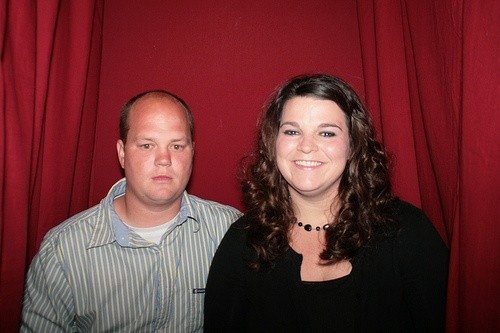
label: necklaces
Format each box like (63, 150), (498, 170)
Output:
(290, 217), (337, 232)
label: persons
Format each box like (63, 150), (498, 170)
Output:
(202, 74), (449, 333)
(19, 90), (245, 333)
